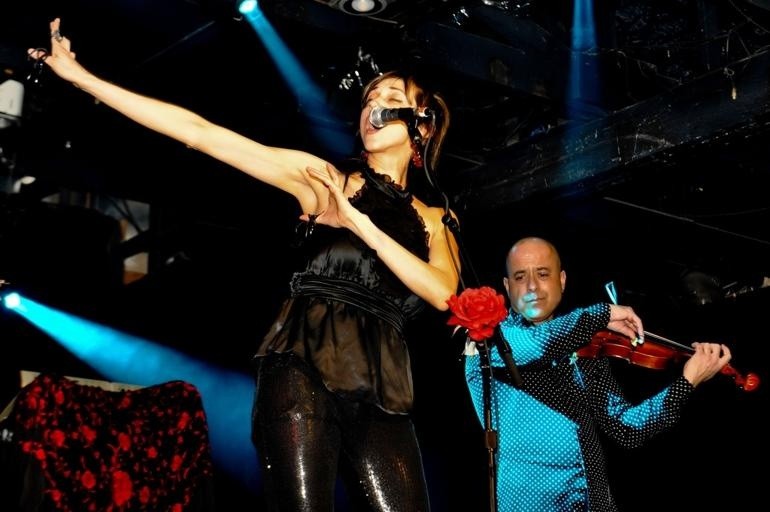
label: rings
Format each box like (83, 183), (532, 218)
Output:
(50, 29), (62, 43)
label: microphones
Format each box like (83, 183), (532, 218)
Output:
(369, 106), (432, 128)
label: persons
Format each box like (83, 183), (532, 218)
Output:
(462, 235), (734, 511)
(24, 16), (462, 509)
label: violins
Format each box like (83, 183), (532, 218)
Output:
(577, 329), (761, 391)
